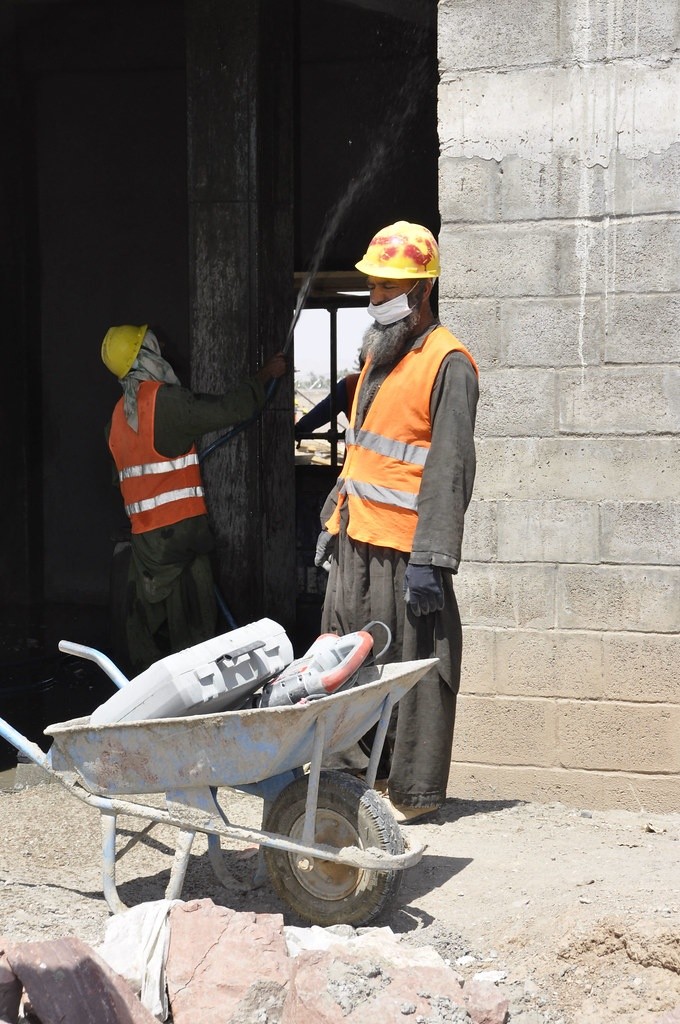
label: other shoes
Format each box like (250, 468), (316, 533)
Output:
(386, 801), (442, 822)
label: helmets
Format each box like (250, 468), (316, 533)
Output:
(355, 221), (441, 278)
(100, 325), (150, 380)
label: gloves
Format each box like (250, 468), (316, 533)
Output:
(404, 562), (444, 615)
(313, 529), (336, 572)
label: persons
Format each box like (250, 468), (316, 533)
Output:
(313, 218), (481, 827)
(99, 322), (293, 682)
(295, 348), (367, 461)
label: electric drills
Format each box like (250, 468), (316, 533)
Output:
(232, 632), (374, 708)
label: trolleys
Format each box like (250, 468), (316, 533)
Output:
(0, 639), (443, 928)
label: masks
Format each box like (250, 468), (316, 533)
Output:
(366, 282), (422, 325)
